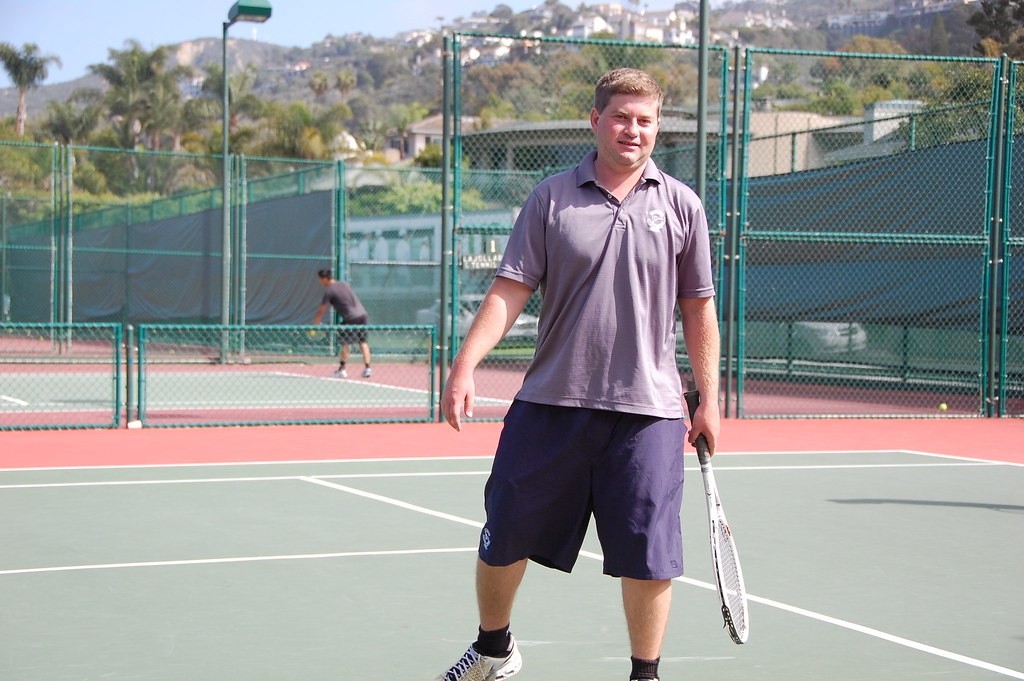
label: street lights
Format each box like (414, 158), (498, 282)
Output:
(217, 0), (274, 364)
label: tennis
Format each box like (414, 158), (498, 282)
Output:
(939, 402), (948, 412)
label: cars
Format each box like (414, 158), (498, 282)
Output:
(674, 319), (688, 353)
(780, 321), (869, 358)
(415, 293), (538, 341)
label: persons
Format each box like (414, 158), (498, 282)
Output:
(310, 268), (372, 378)
(437, 68), (721, 681)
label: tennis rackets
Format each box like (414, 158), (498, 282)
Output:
(683, 389), (750, 645)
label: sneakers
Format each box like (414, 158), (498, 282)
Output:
(433, 631), (522, 681)
(361, 368), (371, 377)
(334, 369), (347, 378)
